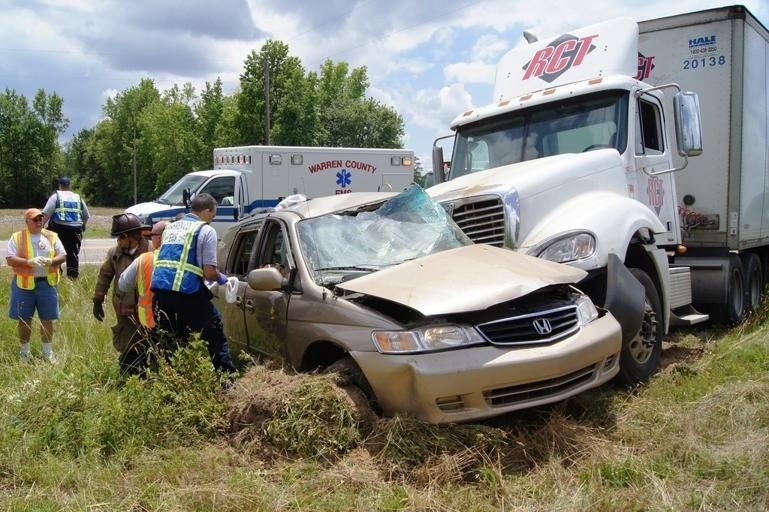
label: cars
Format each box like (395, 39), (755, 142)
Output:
(209, 180), (634, 438)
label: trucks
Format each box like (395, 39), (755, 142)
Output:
(422, 160), (489, 194)
(415, 1), (769, 384)
(117, 143), (416, 244)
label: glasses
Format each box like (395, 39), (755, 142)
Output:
(120, 233), (128, 239)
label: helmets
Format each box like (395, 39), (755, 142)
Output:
(111, 214), (152, 235)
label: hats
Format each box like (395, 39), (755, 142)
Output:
(58, 177), (70, 184)
(24, 207), (44, 219)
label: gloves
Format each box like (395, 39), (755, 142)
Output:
(28, 256), (52, 268)
(218, 272), (227, 284)
(93, 297), (104, 321)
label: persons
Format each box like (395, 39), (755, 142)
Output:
(118, 219), (192, 374)
(4, 206), (68, 363)
(92, 213), (156, 383)
(148, 192), (241, 391)
(41, 175), (91, 281)
